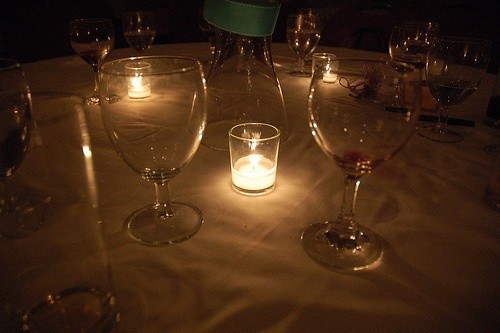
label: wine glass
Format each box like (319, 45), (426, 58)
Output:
(70, 17), (122, 107)
(380, 21), (487, 142)
(99, 55), (207, 247)
(285, 7), (323, 76)
(1, 58), (52, 216)
(301, 58), (421, 273)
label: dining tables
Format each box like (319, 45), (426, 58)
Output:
(0, 41), (500, 333)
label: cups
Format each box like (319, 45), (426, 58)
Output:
(189, 0), (290, 151)
(23, 284), (121, 333)
(124, 62), (152, 100)
(229, 122), (281, 196)
(123, 12), (155, 53)
(312, 53), (338, 83)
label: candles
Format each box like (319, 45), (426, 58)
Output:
(128, 76), (151, 98)
(233, 153), (277, 190)
(323, 64), (337, 82)
